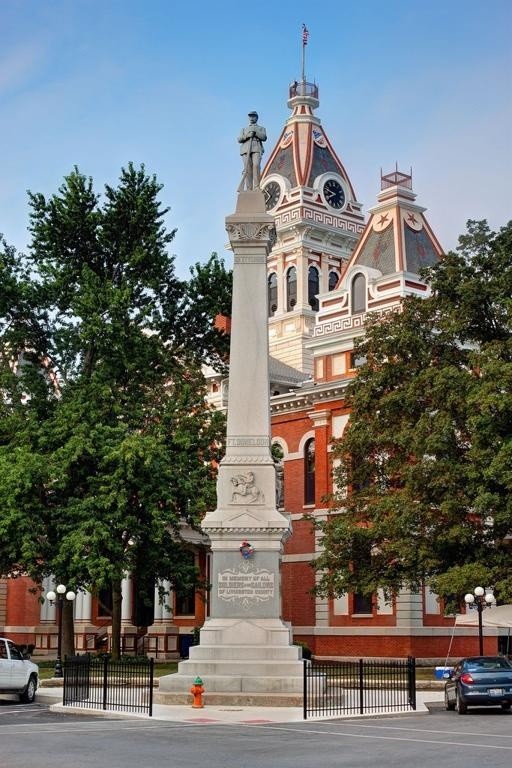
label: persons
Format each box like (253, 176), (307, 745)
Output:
(240, 470), (254, 496)
(238, 110), (267, 191)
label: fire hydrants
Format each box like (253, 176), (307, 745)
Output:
(190, 676), (205, 709)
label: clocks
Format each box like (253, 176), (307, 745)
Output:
(323, 178), (346, 209)
(260, 179), (281, 211)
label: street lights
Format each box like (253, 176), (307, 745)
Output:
(45, 585), (75, 678)
(465, 586), (494, 656)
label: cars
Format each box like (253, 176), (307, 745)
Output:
(444, 655), (512, 714)
(0, 636), (40, 702)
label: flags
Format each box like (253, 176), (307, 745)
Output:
(313, 131), (324, 144)
(302, 26), (309, 45)
